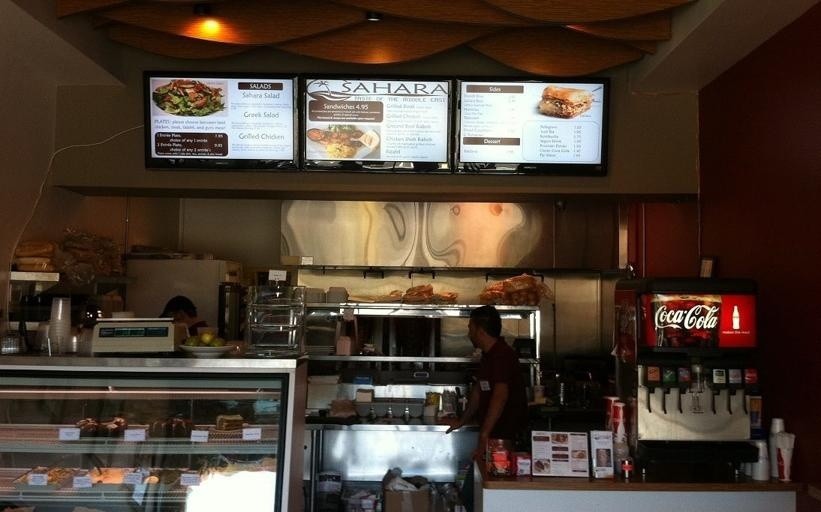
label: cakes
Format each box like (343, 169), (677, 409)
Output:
(75, 417), (128, 438)
(216, 414), (244, 430)
(144, 412), (193, 438)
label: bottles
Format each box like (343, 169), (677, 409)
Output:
(490, 439), (511, 477)
(361, 343), (375, 356)
(440, 483), (471, 512)
(731, 305), (740, 330)
(317, 470), (382, 512)
(429, 482), (437, 511)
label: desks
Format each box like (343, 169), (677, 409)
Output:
(482, 472), (801, 512)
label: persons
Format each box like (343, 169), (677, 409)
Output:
(159, 294), (226, 471)
(445, 305), (528, 511)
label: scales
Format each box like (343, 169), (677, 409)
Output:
(91, 317), (191, 357)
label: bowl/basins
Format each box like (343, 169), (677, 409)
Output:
(303, 287), (348, 304)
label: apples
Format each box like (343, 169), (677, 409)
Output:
(185, 331), (226, 347)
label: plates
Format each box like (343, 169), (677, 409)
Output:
(304, 345), (334, 355)
(309, 125), (379, 160)
(178, 345), (237, 357)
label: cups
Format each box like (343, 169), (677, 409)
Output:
(48, 296), (91, 355)
(741, 418), (796, 483)
(605, 396), (632, 480)
(650, 293), (722, 347)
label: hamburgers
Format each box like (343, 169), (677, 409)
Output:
(538, 88), (590, 118)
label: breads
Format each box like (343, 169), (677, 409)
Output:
(482, 276), (535, 305)
(392, 284), (459, 304)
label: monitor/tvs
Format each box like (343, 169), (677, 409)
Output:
(453, 75), (611, 176)
(142, 69), (300, 172)
(298, 71), (455, 175)
